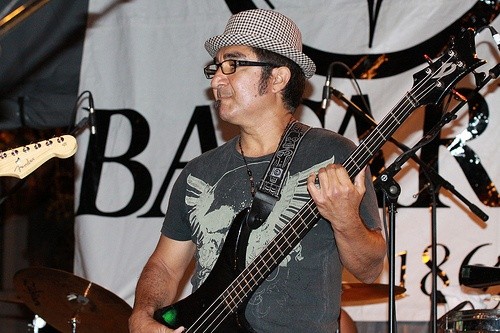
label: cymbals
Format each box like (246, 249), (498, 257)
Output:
(12, 267), (134, 333)
(336, 282), (406, 306)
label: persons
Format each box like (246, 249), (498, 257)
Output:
(129, 9), (386, 333)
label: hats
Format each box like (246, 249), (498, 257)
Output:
(204, 8), (317, 79)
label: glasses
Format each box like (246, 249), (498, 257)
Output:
(204, 60), (284, 79)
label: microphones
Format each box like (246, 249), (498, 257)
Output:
(89, 93), (97, 134)
(321, 66), (332, 109)
(437, 302), (468, 329)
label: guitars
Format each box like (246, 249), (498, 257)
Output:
(1, 132), (77, 181)
(152, 28), (487, 332)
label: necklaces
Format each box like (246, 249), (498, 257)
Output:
(238, 134), (254, 197)
